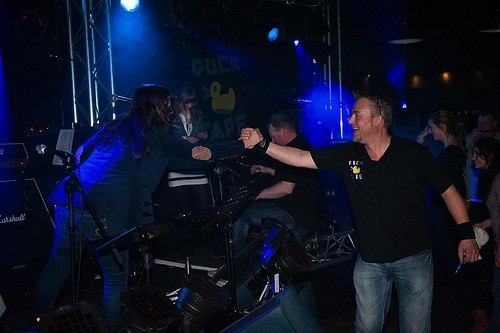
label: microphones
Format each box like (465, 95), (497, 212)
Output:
(110, 94), (133, 104)
(36, 144), (73, 157)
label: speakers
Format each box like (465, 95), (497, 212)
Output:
(220, 285), (324, 333)
(0, 177), (56, 272)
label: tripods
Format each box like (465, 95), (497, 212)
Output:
(304, 192), (357, 264)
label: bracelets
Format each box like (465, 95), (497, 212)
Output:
(253, 138), (269, 154)
(455, 222), (476, 241)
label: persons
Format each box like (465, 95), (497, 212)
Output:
(417, 108), (500, 333)
(163, 100), (214, 221)
(19, 83), (264, 333)
(214, 111), (324, 270)
(240, 91), (481, 333)
